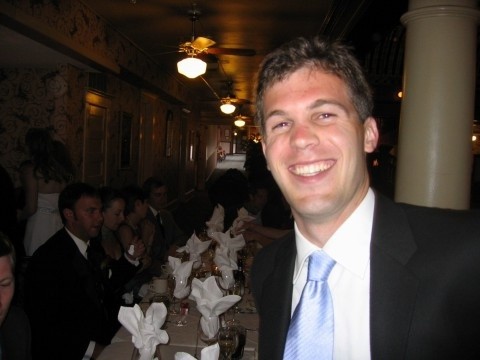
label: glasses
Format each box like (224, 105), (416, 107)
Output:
(152, 192), (169, 197)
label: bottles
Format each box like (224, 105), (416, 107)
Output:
(235, 258), (245, 285)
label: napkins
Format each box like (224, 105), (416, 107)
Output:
(117, 301), (170, 360)
(167, 202), (256, 337)
(173, 342), (221, 360)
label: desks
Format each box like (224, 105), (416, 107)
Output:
(109, 240), (258, 350)
(98, 343), (260, 360)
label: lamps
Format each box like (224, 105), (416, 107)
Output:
(177, 57), (207, 78)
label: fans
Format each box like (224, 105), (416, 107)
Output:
(207, 79), (255, 121)
(150, 7), (257, 57)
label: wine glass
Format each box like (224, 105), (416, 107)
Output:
(218, 327), (239, 360)
(225, 283), (241, 322)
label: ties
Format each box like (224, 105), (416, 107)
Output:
(156, 213), (166, 239)
(282, 251), (337, 360)
(87, 245), (96, 264)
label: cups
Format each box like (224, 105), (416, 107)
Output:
(230, 325), (246, 360)
(148, 293), (172, 310)
(149, 279), (168, 293)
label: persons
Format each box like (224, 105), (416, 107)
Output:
(0, 231), (33, 360)
(249, 35), (480, 360)
(222, 168), (295, 239)
(19, 127), (72, 258)
(26, 180), (139, 360)
(95, 176), (186, 300)
